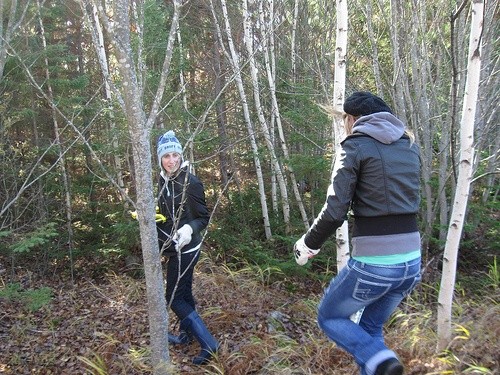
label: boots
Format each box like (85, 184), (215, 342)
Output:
(168, 301), (198, 345)
(182, 311), (220, 365)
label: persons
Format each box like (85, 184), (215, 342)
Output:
(294, 91), (422, 375)
(131, 130), (221, 366)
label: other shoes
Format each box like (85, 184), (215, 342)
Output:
(376, 358), (403, 375)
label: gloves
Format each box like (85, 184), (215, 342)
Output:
(292, 234), (321, 266)
(172, 225), (192, 252)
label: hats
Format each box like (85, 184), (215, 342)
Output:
(344, 91), (393, 116)
(157, 130), (184, 198)
